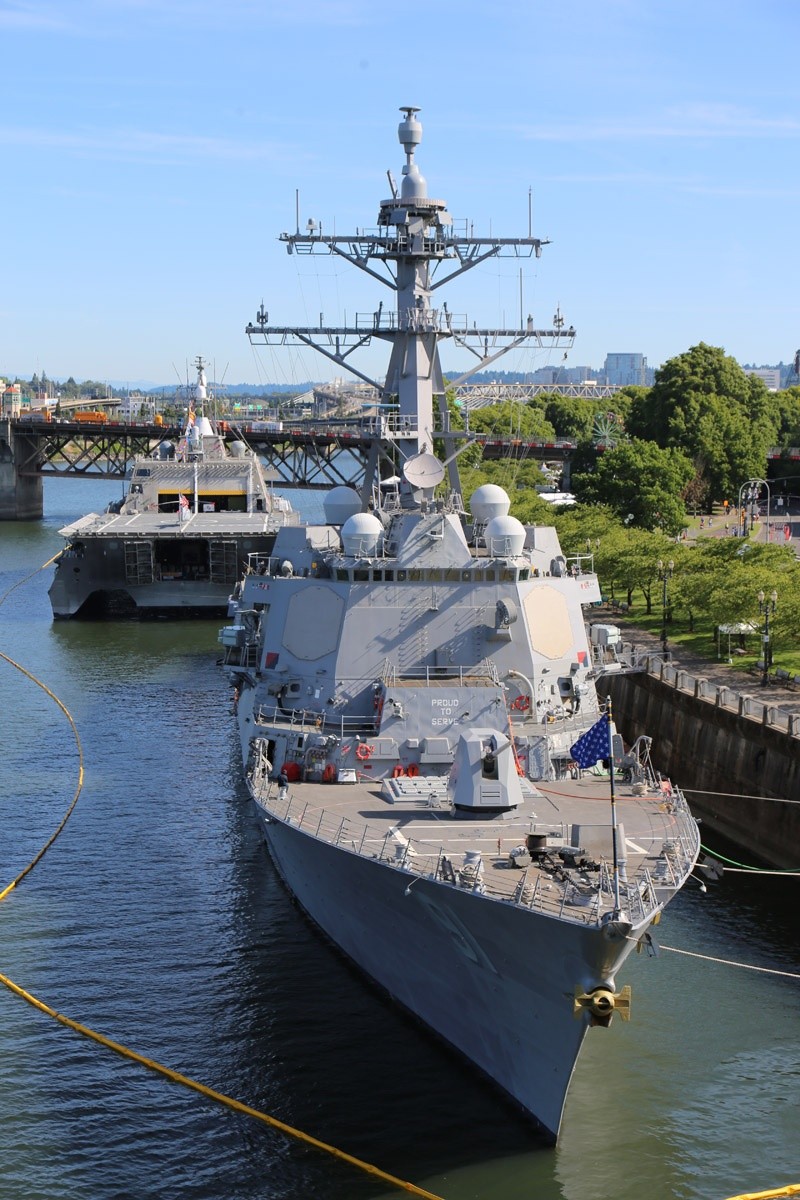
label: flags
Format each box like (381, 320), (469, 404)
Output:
(570, 713), (611, 769)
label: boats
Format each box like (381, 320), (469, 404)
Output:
(42, 354), (301, 620)
(221, 101), (707, 1138)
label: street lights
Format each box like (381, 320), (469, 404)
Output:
(657, 558), (676, 661)
(757, 590), (782, 688)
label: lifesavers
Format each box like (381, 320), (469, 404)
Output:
(356, 745), (371, 760)
(516, 695), (529, 710)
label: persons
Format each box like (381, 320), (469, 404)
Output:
(277, 769), (289, 793)
